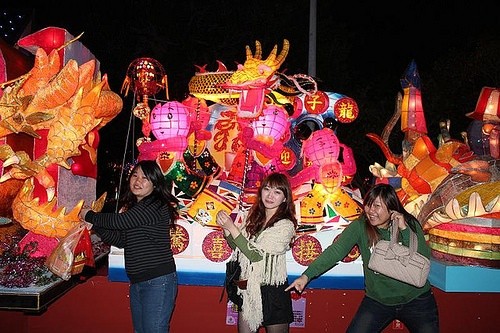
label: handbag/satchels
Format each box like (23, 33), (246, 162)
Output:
(226, 258), (245, 307)
(367, 213), (430, 289)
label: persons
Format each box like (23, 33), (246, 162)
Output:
(216, 172), (297, 333)
(79, 159), (180, 333)
(286, 184), (440, 333)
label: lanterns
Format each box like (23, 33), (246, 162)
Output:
(0, 26), (500, 271)
